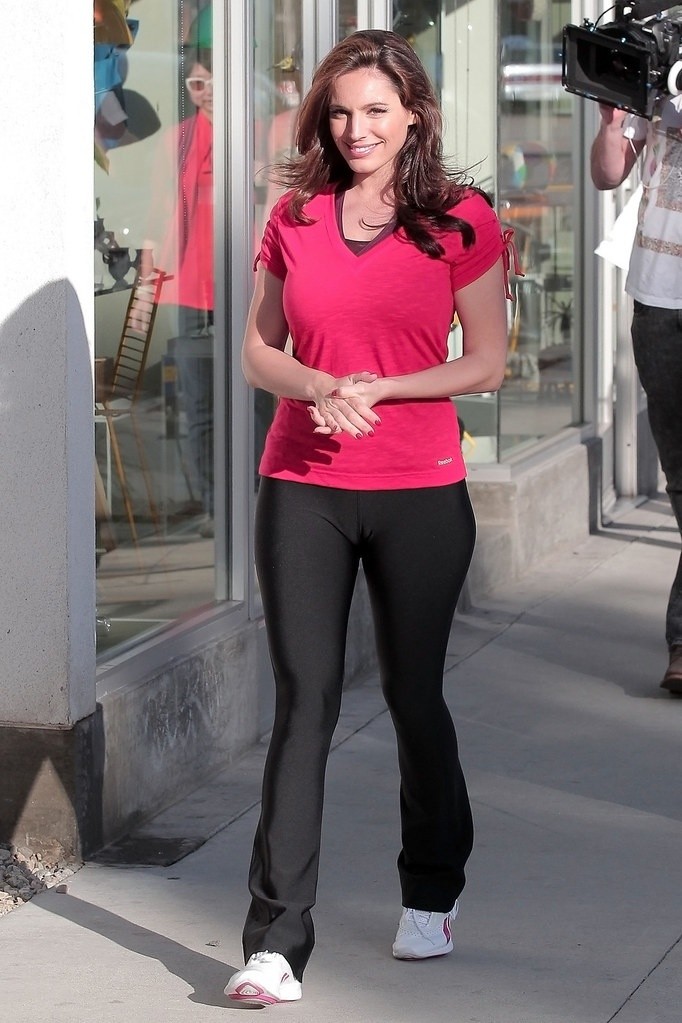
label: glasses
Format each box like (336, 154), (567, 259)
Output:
(185, 78), (214, 93)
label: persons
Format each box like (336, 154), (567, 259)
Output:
(223, 29), (508, 1007)
(128, 44), (265, 541)
(590, 0), (682, 696)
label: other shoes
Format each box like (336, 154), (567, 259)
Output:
(660, 644), (682, 691)
(199, 516), (214, 537)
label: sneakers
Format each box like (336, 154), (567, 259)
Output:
(392, 898), (459, 959)
(224, 951), (302, 1004)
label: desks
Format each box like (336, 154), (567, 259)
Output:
(500, 185), (578, 362)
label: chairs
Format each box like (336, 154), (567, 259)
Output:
(96, 266), (169, 556)
